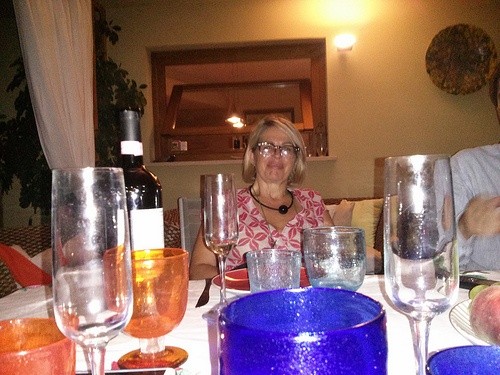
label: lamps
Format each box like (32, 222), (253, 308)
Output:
(224, 63), (246, 128)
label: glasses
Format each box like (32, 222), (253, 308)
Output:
(257, 141), (300, 158)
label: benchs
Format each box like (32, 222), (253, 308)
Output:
(0, 196), (386, 299)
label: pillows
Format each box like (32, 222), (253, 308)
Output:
(352, 198), (384, 248)
(332, 200), (355, 226)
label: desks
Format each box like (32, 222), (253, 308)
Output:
(75, 275), (474, 375)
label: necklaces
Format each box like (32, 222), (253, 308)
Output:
(249, 186), (293, 214)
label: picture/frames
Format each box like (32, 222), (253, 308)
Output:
(244, 107), (295, 125)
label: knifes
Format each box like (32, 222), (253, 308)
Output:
(195, 275), (212, 309)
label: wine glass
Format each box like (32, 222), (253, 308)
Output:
(50, 163), (135, 375)
(114, 244), (192, 369)
(202, 172), (236, 323)
(382, 150), (462, 375)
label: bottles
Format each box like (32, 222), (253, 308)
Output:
(112, 100), (165, 321)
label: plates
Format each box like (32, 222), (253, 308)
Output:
(425, 344), (500, 374)
(457, 268), (500, 291)
(211, 266), (328, 293)
(449, 294), (493, 348)
(423, 20), (495, 95)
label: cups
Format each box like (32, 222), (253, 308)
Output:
(307, 132), (328, 158)
(217, 286), (390, 375)
(246, 249), (302, 295)
(0, 315), (78, 375)
(302, 225), (365, 291)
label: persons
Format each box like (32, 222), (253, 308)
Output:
(435, 72), (499, 272)
(189, 118), (341, 281)
